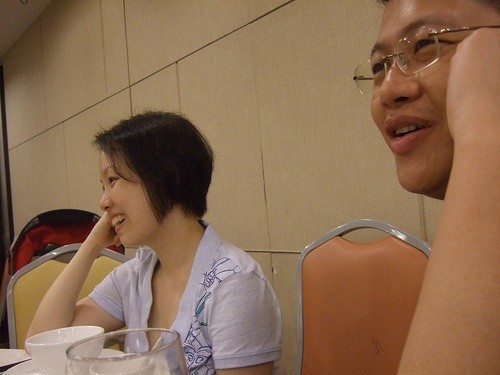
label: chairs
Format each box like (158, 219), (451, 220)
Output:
(295, 219), (431, 375)
(7, 242), (130, 349)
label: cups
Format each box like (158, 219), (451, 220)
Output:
(65, 328), (188, 375)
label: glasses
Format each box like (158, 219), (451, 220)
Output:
(353, 25), (500, 98)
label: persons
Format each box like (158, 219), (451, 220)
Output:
(25, 111), (283, 375)
(352, 0), (500, 375)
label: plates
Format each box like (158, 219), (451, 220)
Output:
(0, 348), (125, 375)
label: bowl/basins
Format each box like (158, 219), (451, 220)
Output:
(25, 326), (104, 375)
